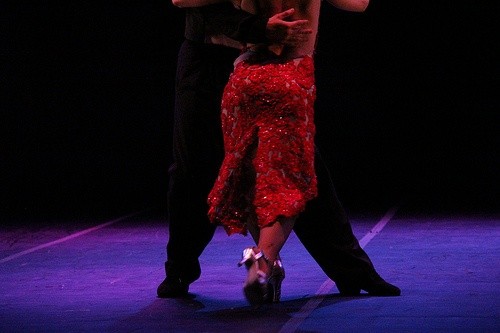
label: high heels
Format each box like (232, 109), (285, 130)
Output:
(237, 248), (267, 312)
(269, 259), (285, 307)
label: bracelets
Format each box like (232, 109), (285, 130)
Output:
(261, 15), (268, 31)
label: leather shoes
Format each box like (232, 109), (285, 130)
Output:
(157, 275), (188, 300)
(336, 280), (402, 297)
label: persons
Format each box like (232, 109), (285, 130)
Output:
(170, 0), (369, 307)
(157, 0), (401, 298)
